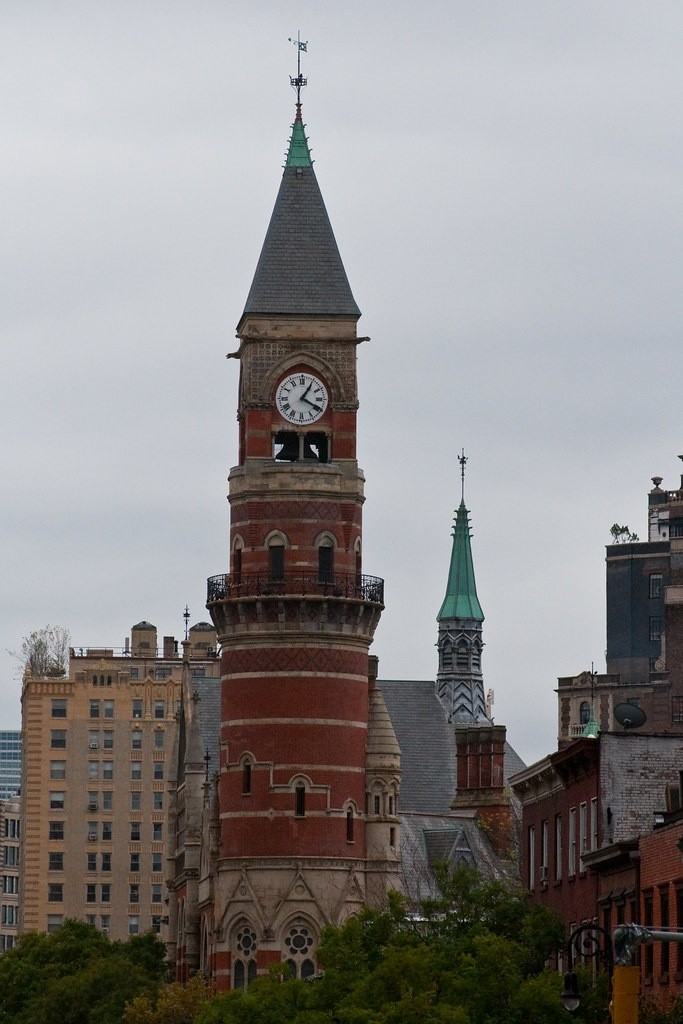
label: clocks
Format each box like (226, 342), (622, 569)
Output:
(275, 373), (328, 425)
